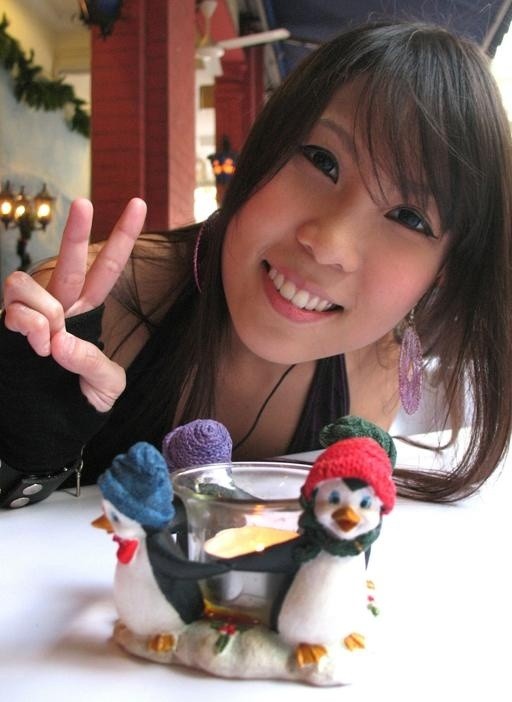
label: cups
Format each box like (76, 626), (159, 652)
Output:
(170, 462), (314, 629)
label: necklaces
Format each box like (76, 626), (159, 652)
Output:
(203, 362), (300, 469)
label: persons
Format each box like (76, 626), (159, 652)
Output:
(0, 18), (509, 515)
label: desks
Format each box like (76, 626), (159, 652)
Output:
(0, 424), (512, 702)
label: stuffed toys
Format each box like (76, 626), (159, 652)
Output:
(319, 416), (397, 618)
(91, 442), (230, 654)
(217, 437), (397, 666)
(161, 419), (246, 561)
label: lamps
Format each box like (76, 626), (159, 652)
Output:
(206, 129), (237, 182)
(0, 178), (64, 274)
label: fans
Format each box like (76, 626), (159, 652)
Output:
(195, 0), (291, 77)
